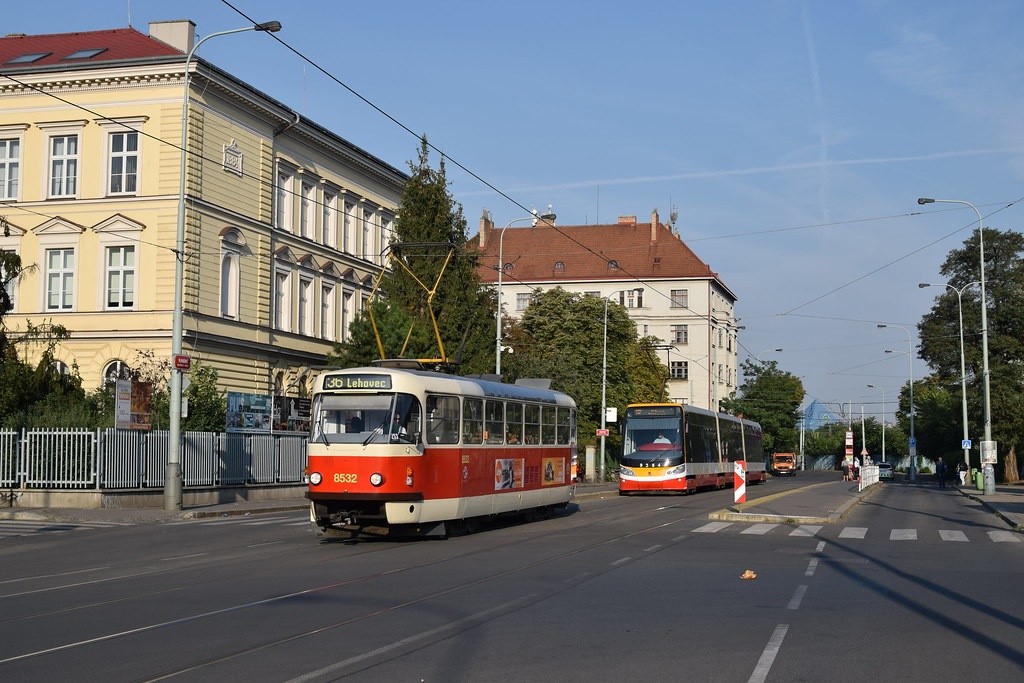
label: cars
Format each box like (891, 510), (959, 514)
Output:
(875, 462), (895, 481)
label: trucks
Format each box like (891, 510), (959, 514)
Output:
(770, 452), (797, 477)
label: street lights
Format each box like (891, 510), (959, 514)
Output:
(599, 288), (645, 483)
(866, 384), (886, 463)
(878, 324), (916, 482)
(756, 349), (782, 384)
(165, 21), (282, 514)
(919, 278), (1000, 486)
(495, 212), (557, 376)
(919, 197), (996, 497)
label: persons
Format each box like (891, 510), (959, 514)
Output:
(383, 413), (406, 434)
(653, 431), (671, 443)
(350, 416), (364, 432)
(936, 457), (948, 487)
(957, 459), (968, 486)
(841, 456), (874, 481)
(506, 425), (521, 444)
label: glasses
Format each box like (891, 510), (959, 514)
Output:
(394, 418), (399, 420)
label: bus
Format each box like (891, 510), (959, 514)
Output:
(303, 358), (578, 542)
(616, 402), (767, 496)
